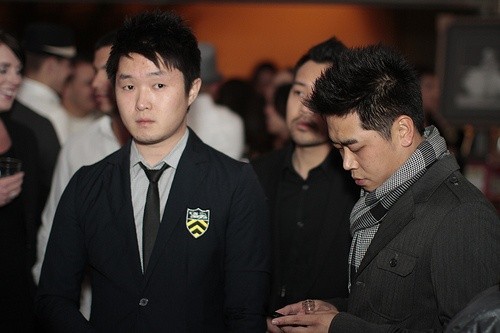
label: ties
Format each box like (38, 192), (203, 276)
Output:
(138, 161), (171, 273)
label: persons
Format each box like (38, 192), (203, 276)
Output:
(268, 43), (500, 333)
(0, 26), (500, 333)
(34, 13), (267, 333)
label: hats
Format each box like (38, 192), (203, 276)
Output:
(33, 24), (76, 58)
